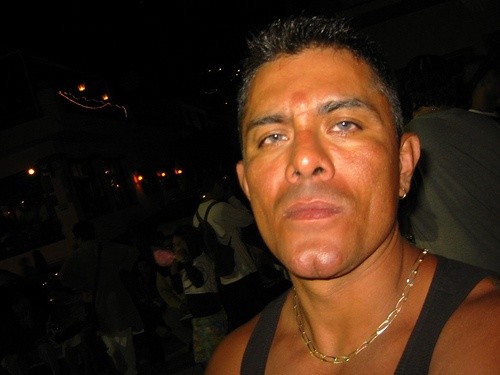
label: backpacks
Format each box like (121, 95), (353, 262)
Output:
(196, 199), (235, 271)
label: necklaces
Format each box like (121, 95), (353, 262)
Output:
(275, 244), (430, 368)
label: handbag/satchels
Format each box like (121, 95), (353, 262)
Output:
(74, 301), (101, 332)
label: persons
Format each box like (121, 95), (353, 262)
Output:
(0, 160), (274, 375)
(197, 17), (500, 375)
